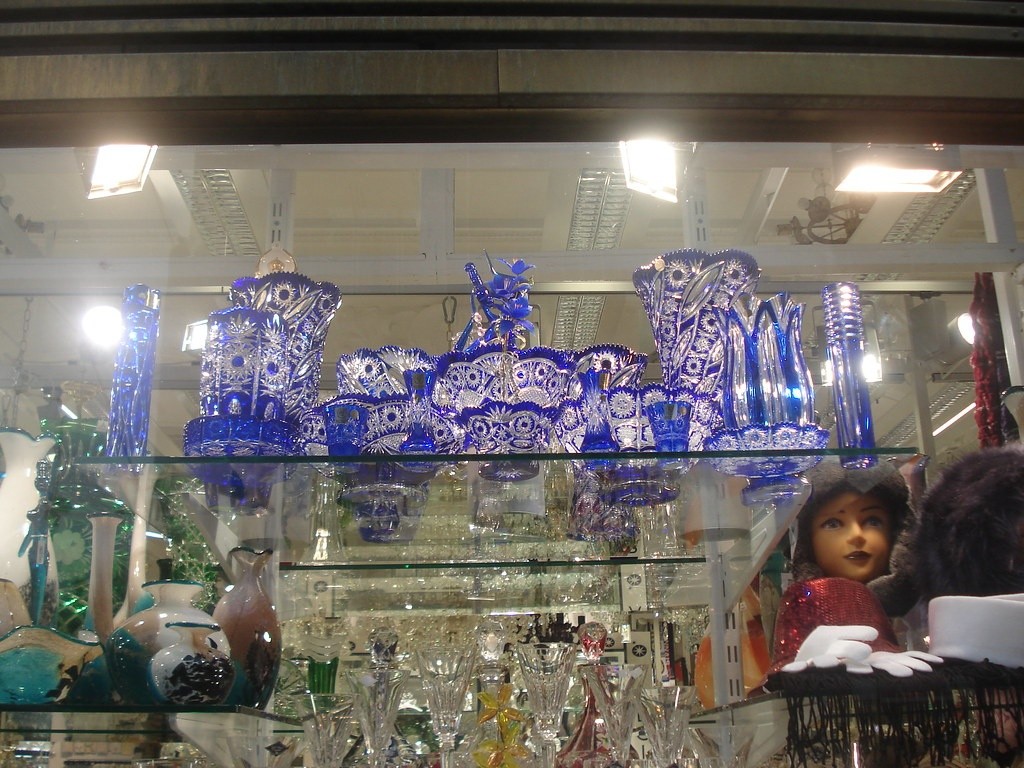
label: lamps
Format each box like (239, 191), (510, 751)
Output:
(71, 144), (158, 201)
(181, 316), (207, 354)
(621, 137), (699, 204)
(81, 294), (129, 350)
(831, 142), (962, 195)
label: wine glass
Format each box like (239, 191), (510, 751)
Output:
(226, 640), (696, 768)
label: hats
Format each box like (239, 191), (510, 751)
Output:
(767, 577), (904, 674)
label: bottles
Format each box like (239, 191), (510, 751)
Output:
(66, 462), (282, 713)
(0, 427), (63, 633)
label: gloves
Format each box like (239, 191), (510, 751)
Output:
(781, 625), (878, 674)
(870, 651), (945, 678)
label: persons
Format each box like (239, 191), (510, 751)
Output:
(805, 477), (902, 580)
